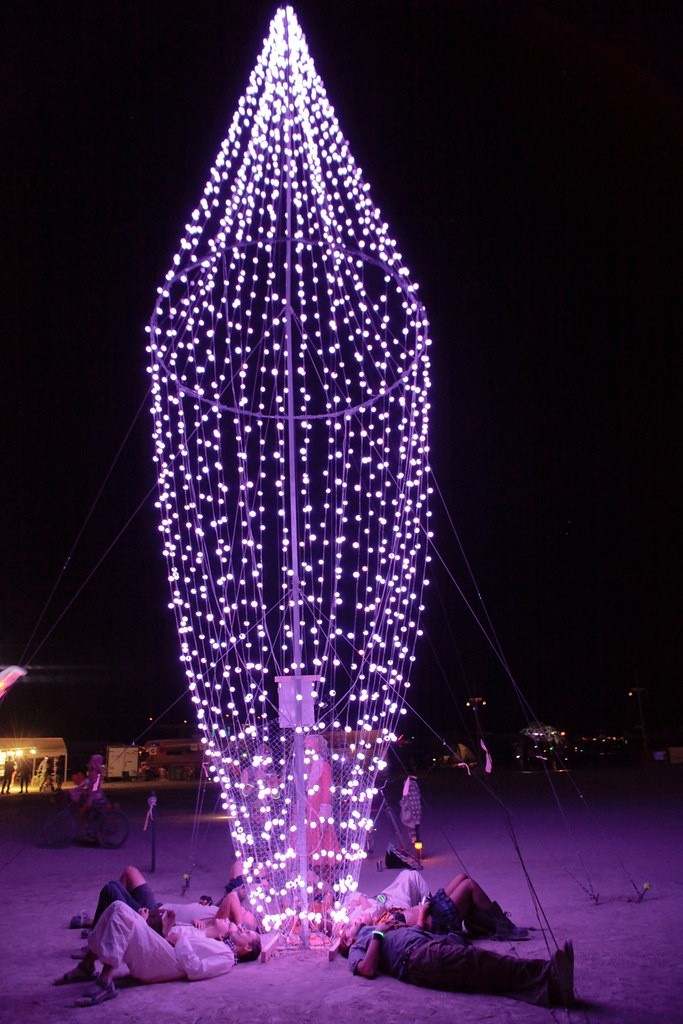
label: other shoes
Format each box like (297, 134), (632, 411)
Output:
(69, 908), (89, 929)
(80, 928), (92, 938)
(549, 937), (575, 1007)
(70, 944), (87, 958)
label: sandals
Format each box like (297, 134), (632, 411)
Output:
(74, 978), (119, 1007)
(63, 962), (99, 982)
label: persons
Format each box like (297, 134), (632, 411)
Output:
(54, 733), (574, 1012)
(2, 756), (105, 842)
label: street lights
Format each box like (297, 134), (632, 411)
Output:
(627, 686), (650, 757)
(465, 696), (488, 757)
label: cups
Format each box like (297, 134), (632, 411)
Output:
(377, 860), (382, 872)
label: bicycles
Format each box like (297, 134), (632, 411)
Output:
(43, 786), (131, 849)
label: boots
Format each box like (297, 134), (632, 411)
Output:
(482, 901), (532, 940)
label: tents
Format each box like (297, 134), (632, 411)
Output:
(0, 737), (67, 785)
